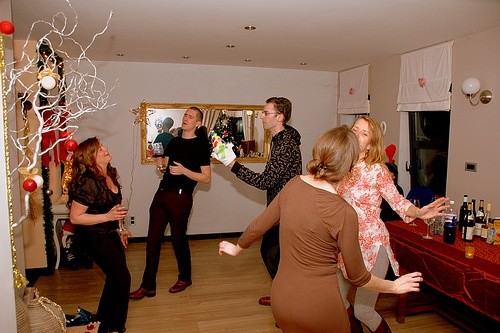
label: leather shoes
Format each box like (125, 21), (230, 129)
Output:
(168, 278), (193, 294)
(129, 287), (157, 300)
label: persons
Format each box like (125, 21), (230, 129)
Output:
(218, 125), (423, 333)
(130, 106), (211, 300)
(65, 136), (133, 333)
(211, 96), (303, 329)
(335, 115), (450, 333)
(153, 117), (207, 155)
(380, 162), (405, 222)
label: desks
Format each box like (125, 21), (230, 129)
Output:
(385, 215), (500, 324)
(51, 204), (70, 270)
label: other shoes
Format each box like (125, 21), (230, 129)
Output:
(259, 297), (271, 306)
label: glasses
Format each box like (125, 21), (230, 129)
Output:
(263, 111), (278, 116)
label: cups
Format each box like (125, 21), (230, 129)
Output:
(464, 242), (475, 259)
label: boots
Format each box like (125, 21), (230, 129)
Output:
(373, 315), (392, 333)
(347, 302), (365, 333)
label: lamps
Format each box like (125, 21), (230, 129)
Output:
(460, 78), (493, 107)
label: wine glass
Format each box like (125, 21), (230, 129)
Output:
(421, 218), (435, 239)
(410, 199), (420, 226)
(152, 142), (166, 170)
(116, 196), (129, 232)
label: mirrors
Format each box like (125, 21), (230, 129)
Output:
(139, 101), (272, 167)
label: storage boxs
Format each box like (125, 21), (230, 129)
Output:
(493, 217), (500, 245)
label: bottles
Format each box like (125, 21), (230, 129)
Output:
(431, 195), (500, 245)
